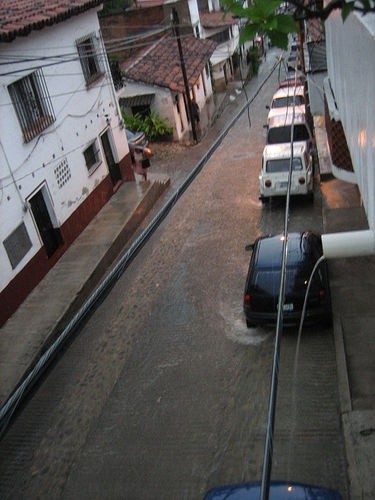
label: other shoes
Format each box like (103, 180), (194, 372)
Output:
(140, 180), (147, 184)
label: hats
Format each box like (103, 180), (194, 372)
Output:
(135, 145), (144, 149)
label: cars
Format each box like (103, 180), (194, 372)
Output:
(259, 74), (312, 211)
(126, 130), (148, 153)
(204, 482), (339, 500)
(288, 33), (300, 71)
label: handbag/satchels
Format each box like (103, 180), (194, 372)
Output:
(142, 153), (150, 168)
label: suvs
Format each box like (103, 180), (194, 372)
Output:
(242, 230), (328, 330)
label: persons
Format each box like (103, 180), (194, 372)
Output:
(191, 98), (200, 124)
